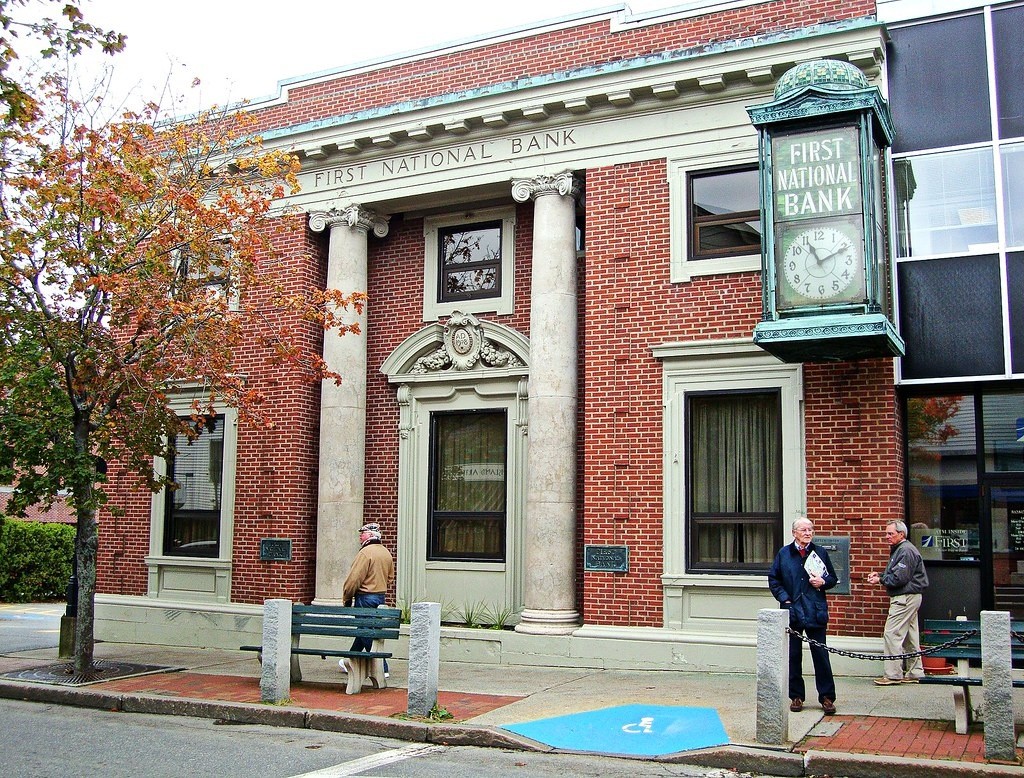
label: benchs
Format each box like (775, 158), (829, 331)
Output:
(239, 602), (401, 695)
(917, 619), (1024, 734)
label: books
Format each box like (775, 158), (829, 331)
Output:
(804, 549), (830, 592)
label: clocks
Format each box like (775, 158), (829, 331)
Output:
(774, 213), (868, 310)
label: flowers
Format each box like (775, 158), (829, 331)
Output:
(919, 628), (951, 646)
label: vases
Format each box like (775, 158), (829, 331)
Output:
(920, 645), (945, 667)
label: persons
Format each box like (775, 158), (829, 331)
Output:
(868, 521), (926, 685)
(339, 523), (395, 678)
(768, 518), (839, 715)
(911, 522), (941, 560)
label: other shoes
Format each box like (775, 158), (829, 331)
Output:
(383, 672), (389, 678)
(822, 698), (836, 715)
(900, 677), (920, 683)
(874, 677), (901, 684)
(790, 697), (802, 712)
(339, 658), (347, 672)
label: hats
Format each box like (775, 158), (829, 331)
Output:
(358, 522), (380, 532)
